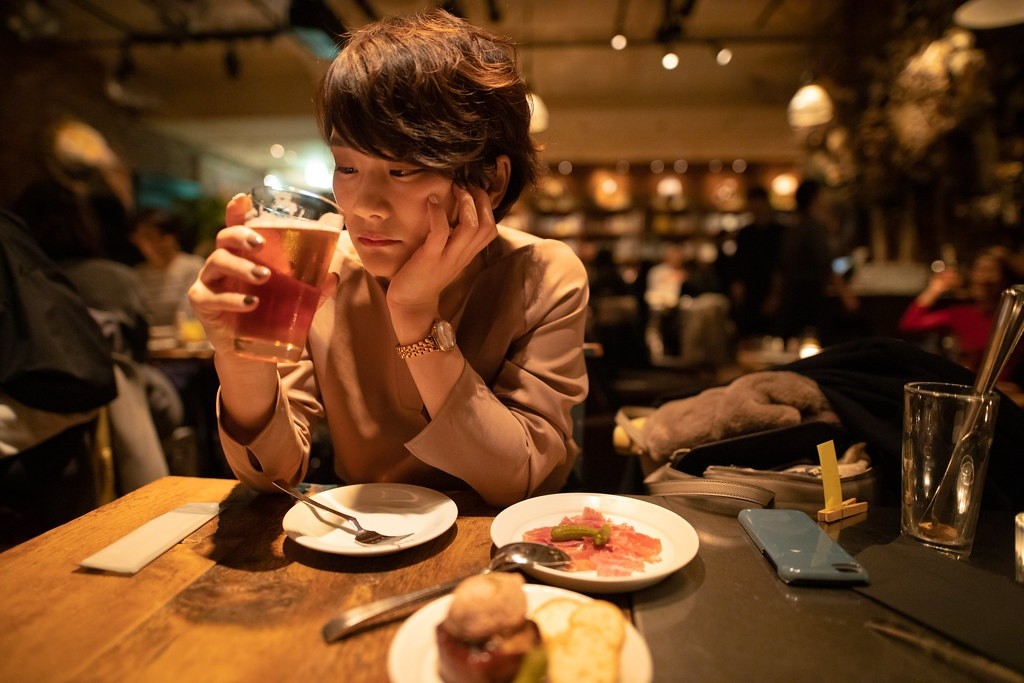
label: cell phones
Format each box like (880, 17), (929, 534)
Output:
(737, 508), (870, 587)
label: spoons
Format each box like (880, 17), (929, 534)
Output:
(323, 543), (575, 644)
(931, 321), (1024, 538)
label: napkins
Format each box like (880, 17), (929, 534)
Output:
(75, 501), (228, 576)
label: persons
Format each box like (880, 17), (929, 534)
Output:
(186, 9), (589, 521)
(129, 208), (204, 394)
(5, 175), (153, 363)
(591, 178), (1024, 383)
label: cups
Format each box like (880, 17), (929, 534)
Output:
(904, 380), (1001, 557)
(232, 184), (344, 363)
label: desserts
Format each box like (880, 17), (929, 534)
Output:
(436, 573), (541, 682)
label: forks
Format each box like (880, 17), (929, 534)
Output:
(272, 478), (414, 546)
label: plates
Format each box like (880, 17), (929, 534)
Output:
(489, 492), (700, 593)
(281, 483), (458, 555)
(384, 582), (654, 683)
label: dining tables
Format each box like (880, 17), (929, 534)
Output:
(0, 475), (1024, 683)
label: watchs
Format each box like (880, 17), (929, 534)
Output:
(395, 318), (457, 360)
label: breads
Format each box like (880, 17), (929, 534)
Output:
(547, 601), (626, 683)
(532, 597), (579, 646)
